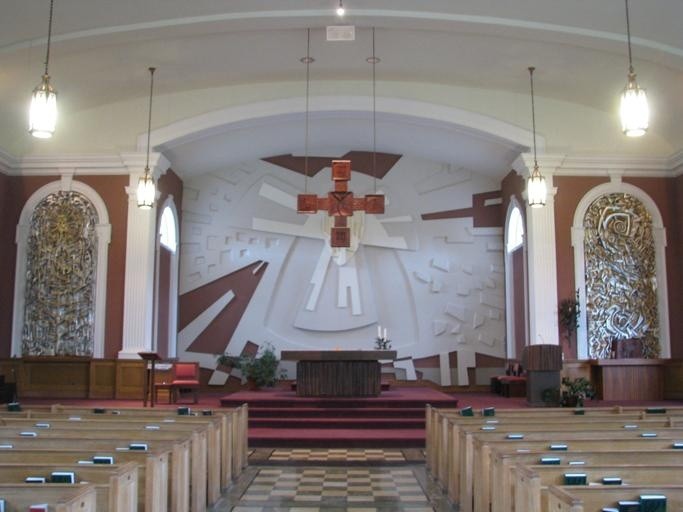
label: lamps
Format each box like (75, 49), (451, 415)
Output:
(525, 67), (549, 207)
(618, 0), (653, 138)
(136, 67), (158, 211)
(28, 0), (60, 140)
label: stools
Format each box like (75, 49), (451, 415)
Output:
(154, 382), (171, 403)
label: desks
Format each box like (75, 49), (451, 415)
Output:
(281, 350), (396, 398)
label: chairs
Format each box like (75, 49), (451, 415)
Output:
(171, 363), (199, 404)
(490, 363), (527, 397)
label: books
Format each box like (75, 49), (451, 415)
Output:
(0, 402), (214, 512)
(458, 406), (683, 512)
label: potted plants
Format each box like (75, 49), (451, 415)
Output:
(219, 339), (279, 391)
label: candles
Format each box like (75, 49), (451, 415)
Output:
(377, 325), (382, 340)
(383, 328), (387, 342)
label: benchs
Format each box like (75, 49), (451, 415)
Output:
(419, 402), (681, 512)
(0, 403), (249, 512)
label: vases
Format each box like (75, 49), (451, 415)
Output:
(566, 399), (578, 407)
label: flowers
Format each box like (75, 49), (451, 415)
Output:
(542, 376), (597, 407)
